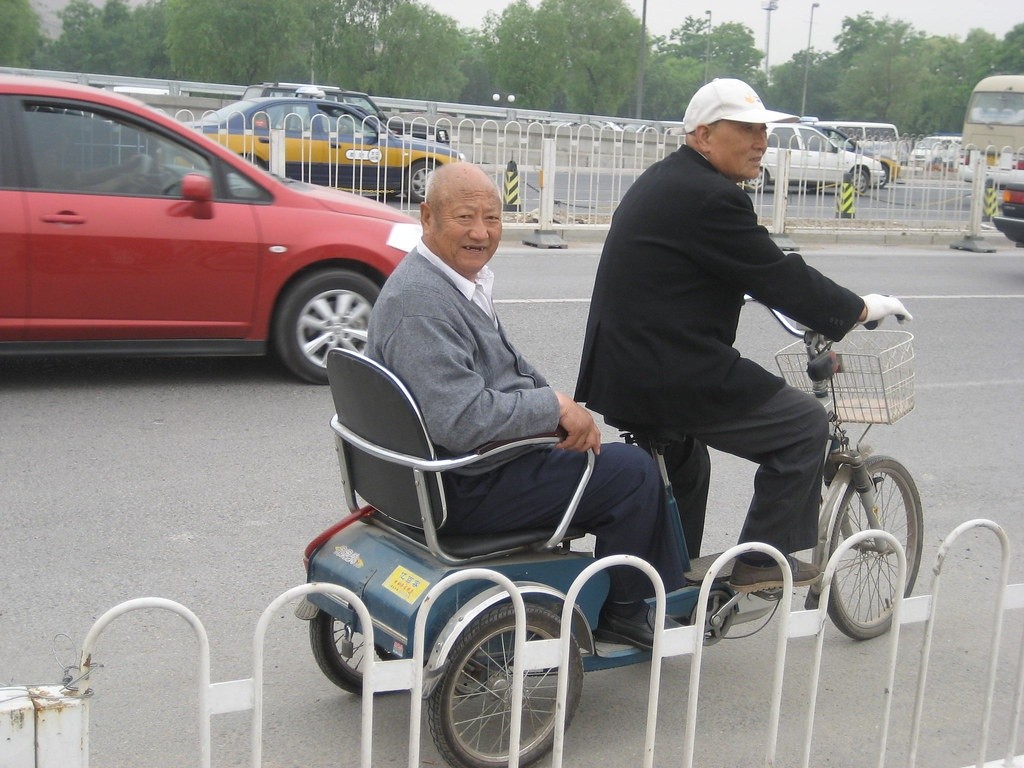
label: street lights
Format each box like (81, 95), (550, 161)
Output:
(761, 0), (778, 108)
(799, 4), (821, 117)
(705, 10), (711, 87)
(635, 0), (646, 119)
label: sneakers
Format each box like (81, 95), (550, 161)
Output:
(729, 553), (823, 595)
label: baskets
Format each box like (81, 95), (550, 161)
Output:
(775, 328), (917, 425)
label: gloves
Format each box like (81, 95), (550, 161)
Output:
(858, 294), (913, 325)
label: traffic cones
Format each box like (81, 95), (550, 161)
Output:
(980, 178), (999, 222)
(835, 172), (855, 218)
(501, 160), (522, 212)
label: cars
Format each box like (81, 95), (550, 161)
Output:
(181, 98), (462, 205)
(993, 160), (1024, 246)
(742, 123), (888, 193)
(806, 124), (902, 191)
(2, 72), (424, 385)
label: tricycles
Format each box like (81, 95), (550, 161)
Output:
(294, 293), (924, 768)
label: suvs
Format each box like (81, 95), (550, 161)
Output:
(243, 83), (451, 147)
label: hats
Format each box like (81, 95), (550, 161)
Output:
(682, 76), (801, 134)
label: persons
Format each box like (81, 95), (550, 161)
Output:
(363, 163), (685, 650)
(28, 110), (153, 192)
(574, 78), (911, 595)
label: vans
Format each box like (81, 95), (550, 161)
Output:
(907, 136), (962, 172)
(800, 121), (899, 183)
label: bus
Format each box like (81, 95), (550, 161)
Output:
(962, 75), (1024, 182)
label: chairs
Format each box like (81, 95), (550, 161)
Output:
(326, 345), (597, 566)
(289, 116), (307, 132)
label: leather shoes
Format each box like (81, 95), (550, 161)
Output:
(595, 603), (690, 653)
